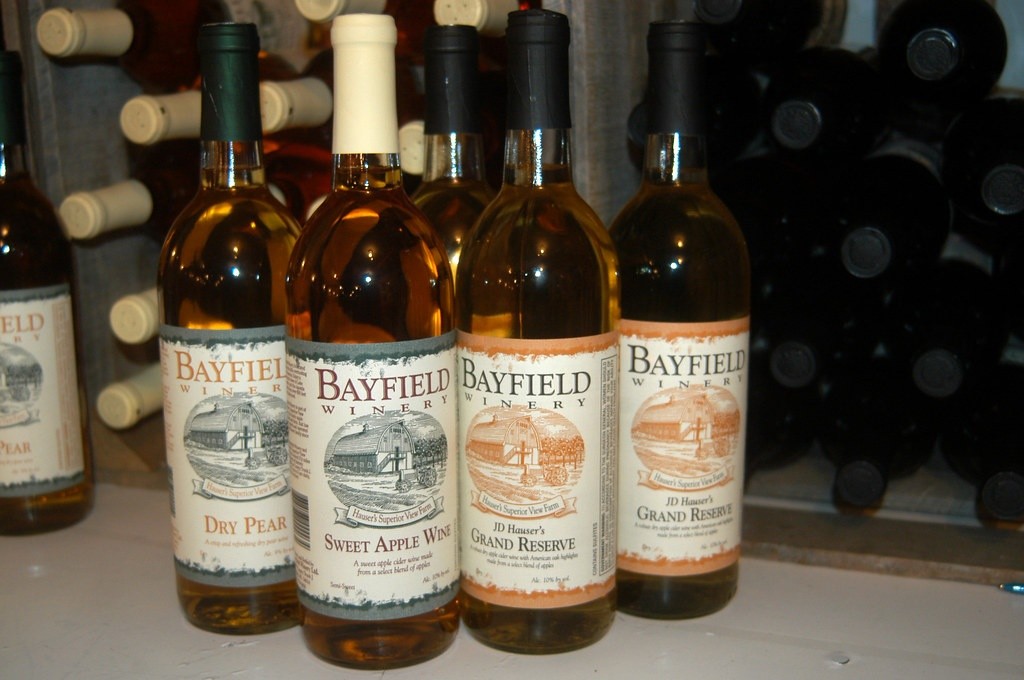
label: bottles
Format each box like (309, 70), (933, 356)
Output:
(406, 22), (493, 305)
(159, 22), (300, 634)
(610, 0), (1023, 620)
(283, 12), (462, 671)
(1, 47), (95, 535)
(35, 0), (537, 432)
(454, 11), (618, 654)
